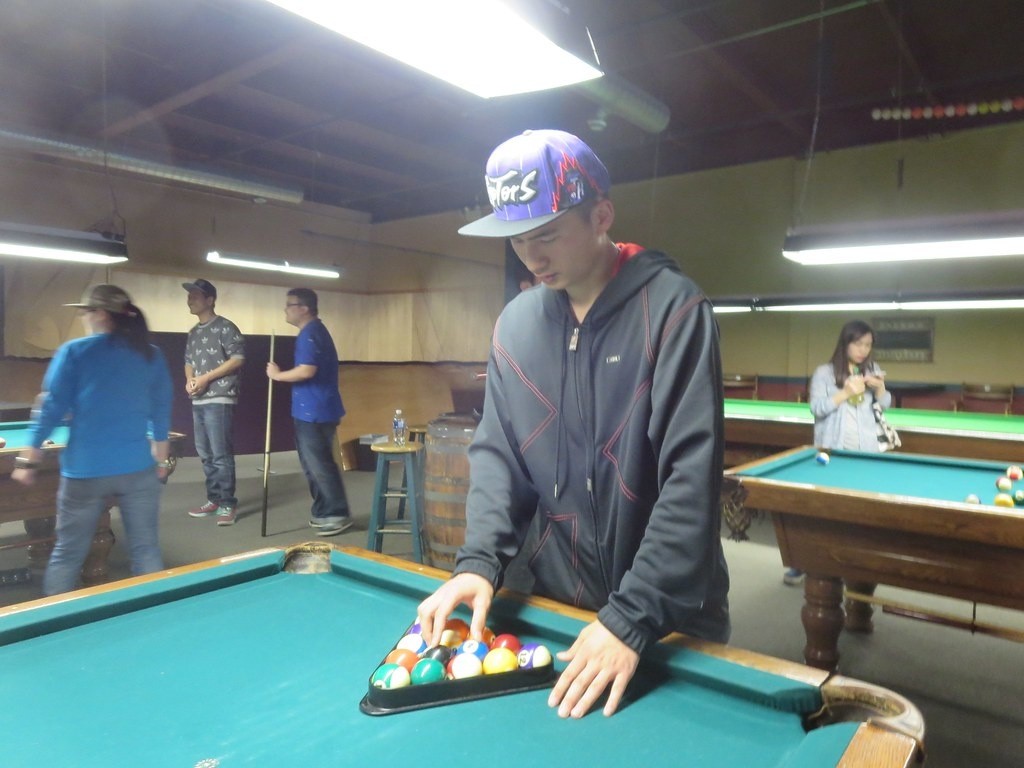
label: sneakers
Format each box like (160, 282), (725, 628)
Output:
(188, 501), (219, 517)
(216, 504), (237, 525)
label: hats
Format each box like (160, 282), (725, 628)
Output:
(182, 279), (217, 300)
(62, 284), (131, 315)
(458, 129), (612, 237)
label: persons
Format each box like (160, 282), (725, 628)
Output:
(416, 129), (731, 721)
(784, 320), (891, 586)
(266, 288), (353, 536)
(11, 285), (174, 597)
(182, 278), (245, 525)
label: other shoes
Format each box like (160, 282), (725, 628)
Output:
(783, 567), (806, 585)
(317, 517), (353, 535)
(309, 520), (327, 527)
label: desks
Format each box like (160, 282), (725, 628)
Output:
(723, 444), (1024, 672)
(720, 397), (1024, 540)
(0, 409), (185, 586)
(0, 538), (926, 768)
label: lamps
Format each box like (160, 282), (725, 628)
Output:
(0, 222), (128, 264)
(705, 1), (1024, 314)
(204, 252), (341, 279)
(280, 0), (605, 98)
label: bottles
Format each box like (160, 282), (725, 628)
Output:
(848, 367), (864, 405)
(392, 410), (407, 448)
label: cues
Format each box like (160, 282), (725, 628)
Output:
(261, 330), (274, 537)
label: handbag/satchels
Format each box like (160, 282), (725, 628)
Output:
(868, 361), (901, 453)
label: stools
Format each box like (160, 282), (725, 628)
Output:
(369, 439), (433, 562)
(396, 424), (428, 517)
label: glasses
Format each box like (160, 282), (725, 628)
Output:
(286, 302), (305, 309)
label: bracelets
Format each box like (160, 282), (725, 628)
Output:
(15, 458), (40, 471)
(157, 460), (168, 467)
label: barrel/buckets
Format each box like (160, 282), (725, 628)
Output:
(423, 417), (484, 572)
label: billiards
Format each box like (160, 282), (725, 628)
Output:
(372, 614), (550, 688)
(964, 465), (1024, 507)
(0, 437), (6, 447)
(43, 440), (55, 444)
(816, 452), (829, 467)
